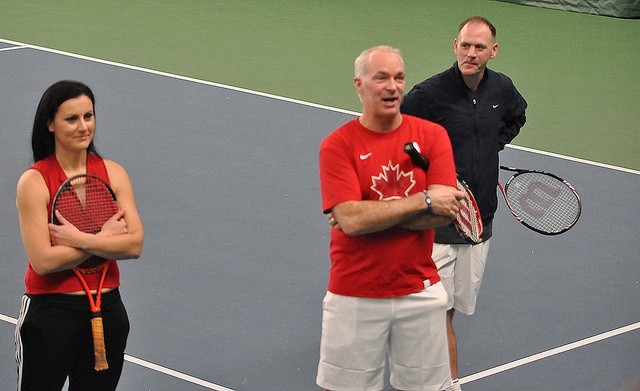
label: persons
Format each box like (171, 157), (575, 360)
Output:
(317, 45), (466, 390)
(399, 17), (529, 391)
(16, 80), (143, 390)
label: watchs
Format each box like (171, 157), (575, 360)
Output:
(422, 190), (432, 210)
